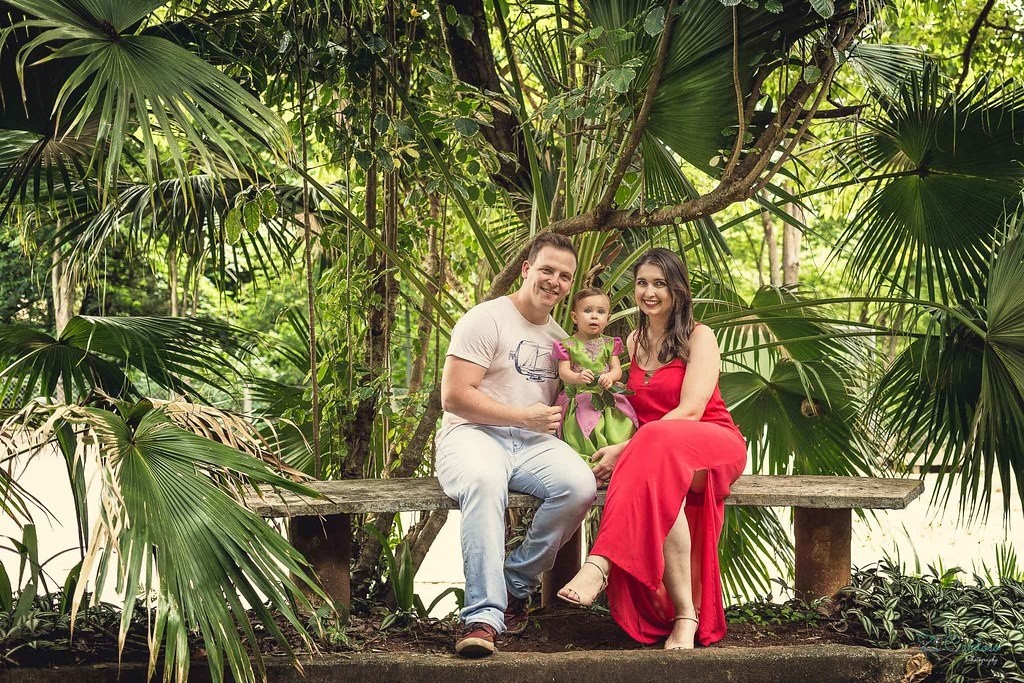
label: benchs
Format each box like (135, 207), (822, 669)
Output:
(234, 474), (925, 622)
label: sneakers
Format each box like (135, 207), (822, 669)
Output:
(455, 622), (498, 658)
(501, 589), (529, 636)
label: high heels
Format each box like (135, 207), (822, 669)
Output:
(664, 607), (699, 650)
(556, 561), (610, 606)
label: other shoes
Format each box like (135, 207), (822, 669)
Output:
(597, 477), (605, 490)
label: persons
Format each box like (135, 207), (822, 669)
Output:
(557, 245), (746, 650)
(550, 288), (639, 488)
(436, 233), (597, 658)
